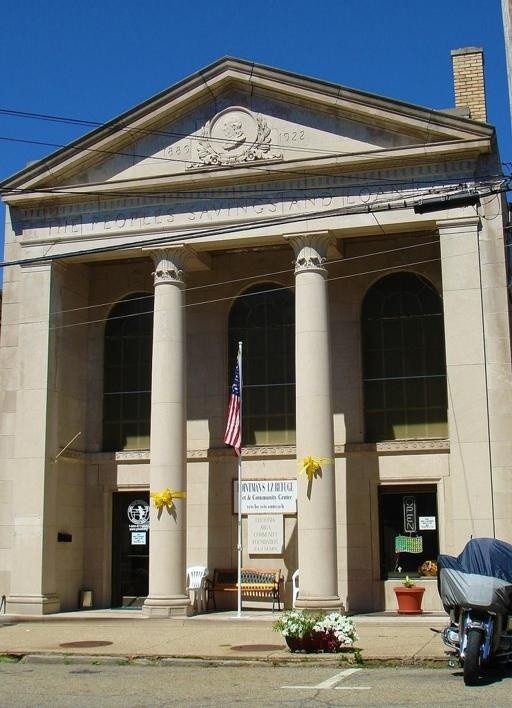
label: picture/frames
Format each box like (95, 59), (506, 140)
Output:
(231, 477), (297, 516)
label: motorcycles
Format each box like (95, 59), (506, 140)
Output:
(429, 608), (512, 686)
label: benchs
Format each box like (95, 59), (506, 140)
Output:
(205, 567), (280, 612)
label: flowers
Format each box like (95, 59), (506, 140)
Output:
(270, 609), (360, 646)
(401, 575), (415, 588)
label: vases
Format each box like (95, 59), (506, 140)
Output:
(285, 633), (339, 652)
(393, 587), (425, 615)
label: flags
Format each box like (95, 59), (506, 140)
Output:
(223, 355), (242, 457)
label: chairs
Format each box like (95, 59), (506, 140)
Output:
(184, 565), (210, 613)
(292, 568), (301, 607)
